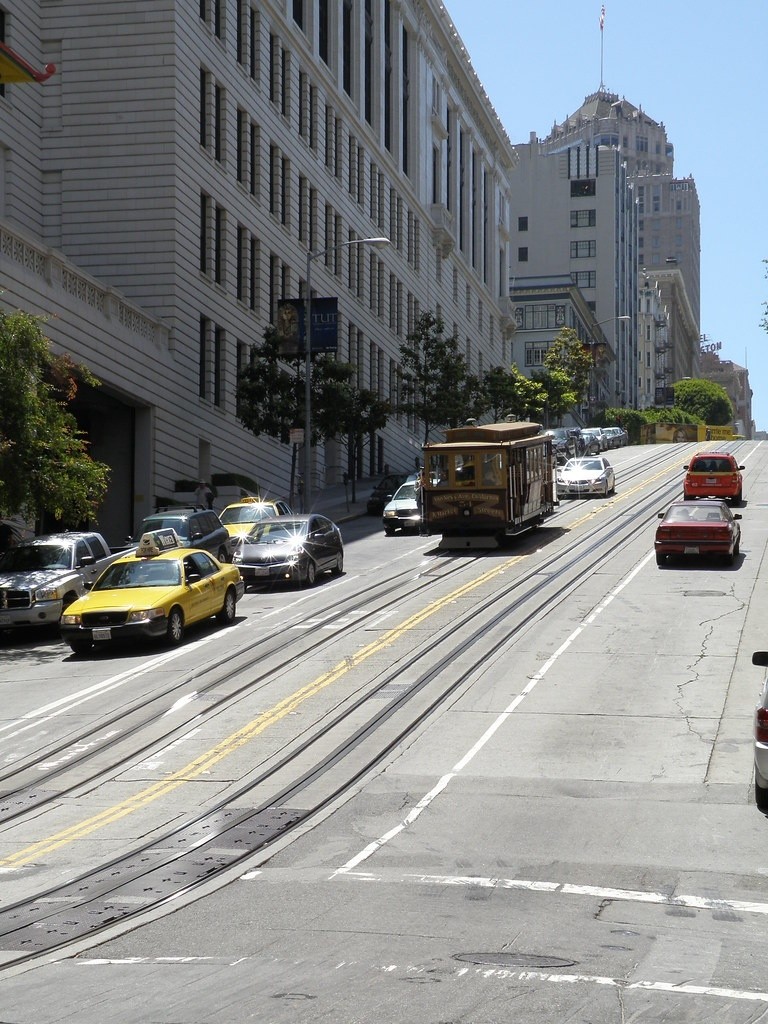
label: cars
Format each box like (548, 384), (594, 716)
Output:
(543, 423), (628, 465)
(367, 473), (415, 516)
(654, 499), (742, 565)
(230, 514), (343, 587)
(214, 497), (296, 544)
(557, 456), (617, 498)
(383, 479), (441, 534)
(57, 528), (246, 650)
(682, 452), (747, 505)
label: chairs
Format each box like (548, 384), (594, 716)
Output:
(706, 513), (721, 522)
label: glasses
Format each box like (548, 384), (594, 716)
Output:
(439, 473), (447, 477)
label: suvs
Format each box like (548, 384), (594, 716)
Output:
(124, 504), (231, 575)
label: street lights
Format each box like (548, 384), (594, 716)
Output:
(303, 236), (390, 516)
(588, 314), (631, 422)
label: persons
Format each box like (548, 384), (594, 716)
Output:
(706, 427), (711, 441)
(673, 428), (688, 443)
(436, 474), (449, 487)
(646, 428), (656, 444)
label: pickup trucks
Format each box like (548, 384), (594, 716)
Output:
(0, 532), (141, 629)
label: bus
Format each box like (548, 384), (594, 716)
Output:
(421, 416), (555, 552)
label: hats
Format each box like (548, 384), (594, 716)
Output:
(198, 479), (206, 484)
